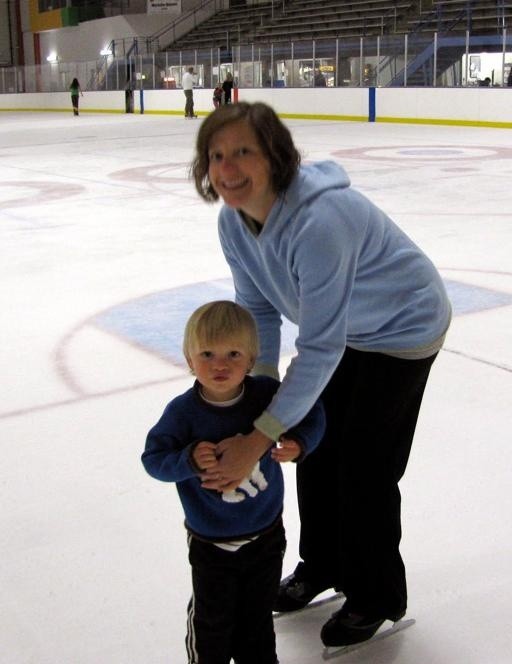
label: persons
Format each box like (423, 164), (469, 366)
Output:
(68, 77), (84, 115)
(313, 69), (327, 88)
(211, 73), (236, 110)
(181, 67), (199, 118)
(140, 301), (326, 663)
(191, 101), (451, 645)
(479, 77), (491, 87)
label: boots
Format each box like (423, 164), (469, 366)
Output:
(320, 597), (407, 647)
(275, 562), (345, 613)
